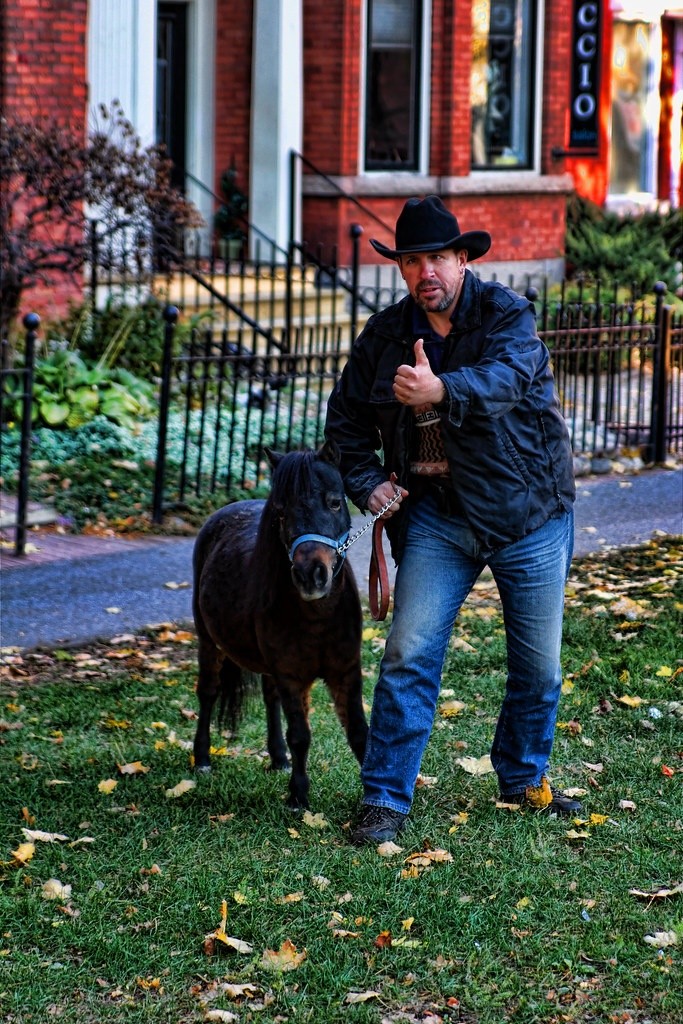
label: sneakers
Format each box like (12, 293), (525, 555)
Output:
(352, 804), (408, 843)
(496, 786), (584, 816)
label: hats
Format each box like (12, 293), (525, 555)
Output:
(370, 195), (492, 263)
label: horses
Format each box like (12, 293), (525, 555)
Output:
(191, 435), (370, 809)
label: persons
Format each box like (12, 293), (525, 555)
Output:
(327, 197), (585, 848)
